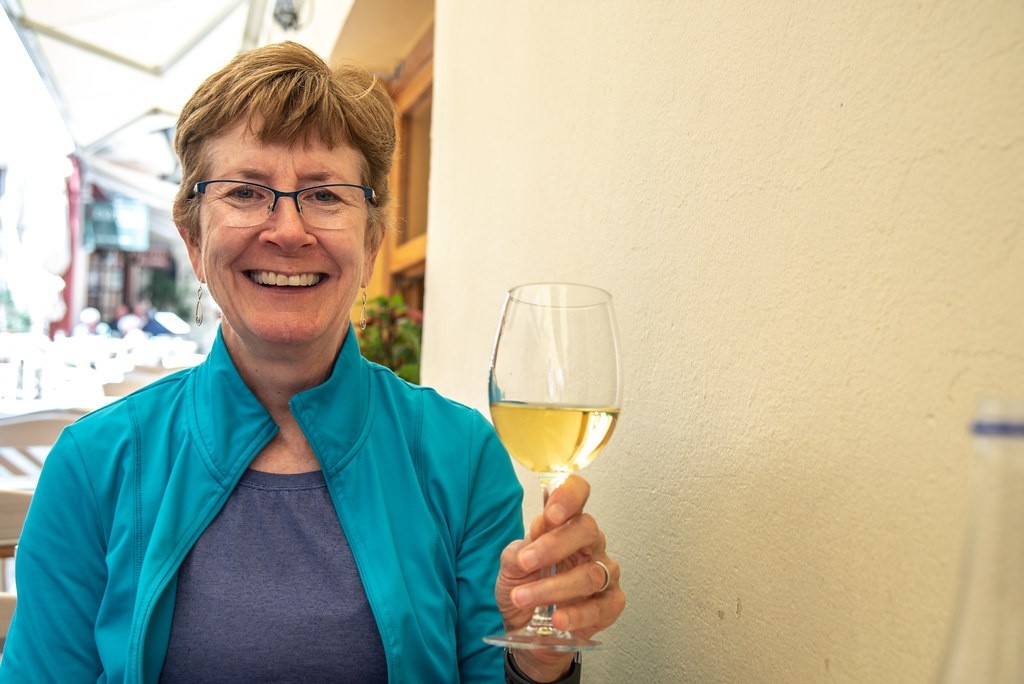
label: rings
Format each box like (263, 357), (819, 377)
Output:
(595, 561), (611, 594)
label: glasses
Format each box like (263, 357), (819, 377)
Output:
(192, 179), (378, 231)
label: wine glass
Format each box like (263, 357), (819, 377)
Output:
(479, 283), (621, 651)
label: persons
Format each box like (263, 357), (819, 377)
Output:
(0, 45), (627, 684)
